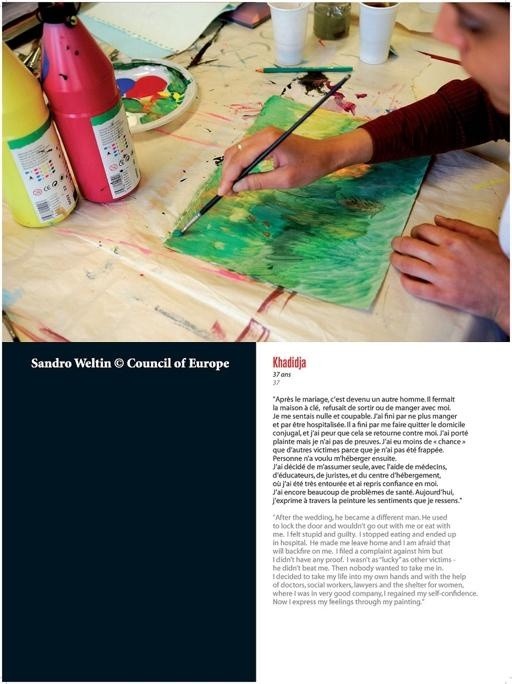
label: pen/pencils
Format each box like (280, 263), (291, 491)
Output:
(255, 65), (353, 73)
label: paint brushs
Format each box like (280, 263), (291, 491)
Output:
(23, 39), (42, 72)
(177, 74), (351, 237)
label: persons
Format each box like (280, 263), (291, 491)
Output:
(217, 2), (509, 337)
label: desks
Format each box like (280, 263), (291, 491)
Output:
(4, 0), (506, 341)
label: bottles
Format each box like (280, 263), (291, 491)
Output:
(313, 2), (352, 41)
(31, 1), (145, 208)
(3, 39), (78, 229)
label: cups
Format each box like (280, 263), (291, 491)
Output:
(266, 3), (310, 66)
(358, 2), (401, 65)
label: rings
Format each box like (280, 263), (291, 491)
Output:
(236, 142), (243, 151)
